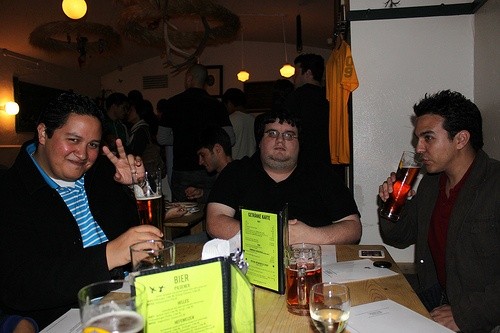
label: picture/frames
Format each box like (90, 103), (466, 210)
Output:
(203, 65), (223, 98)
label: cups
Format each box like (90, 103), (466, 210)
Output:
(284, 243), (324, 316)
(309, 282), (352, 333)
(129, 240), (176, 272)
(380, 151), (425, 222)
(130, 168), (164, 234)
(78, 280), (149, 333)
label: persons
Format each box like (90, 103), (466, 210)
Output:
(374, 89), (500, 333)
(205, 109), (364, 246)
(165, 63), (229, 192)
(183, 124), (232, 200)
(0, 89), (164, 333)
(23, 79), (295, 203)
(253, 54), (343, 178)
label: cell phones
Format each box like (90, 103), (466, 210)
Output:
(359, 250), (386, 258)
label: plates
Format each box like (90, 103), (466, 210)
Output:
(166, 202), (198, 214)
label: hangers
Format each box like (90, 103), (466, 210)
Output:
(334, 27), (344, 51)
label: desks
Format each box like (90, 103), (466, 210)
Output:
(162, 199), (205, 241)
(63, 242), (437, 333)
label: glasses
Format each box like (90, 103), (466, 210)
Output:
(264, 129), (298, 141)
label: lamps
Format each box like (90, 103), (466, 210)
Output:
(236, 26), (249, 82)
(62, 0), (88, 19)
(278, 15), (296, 78)
